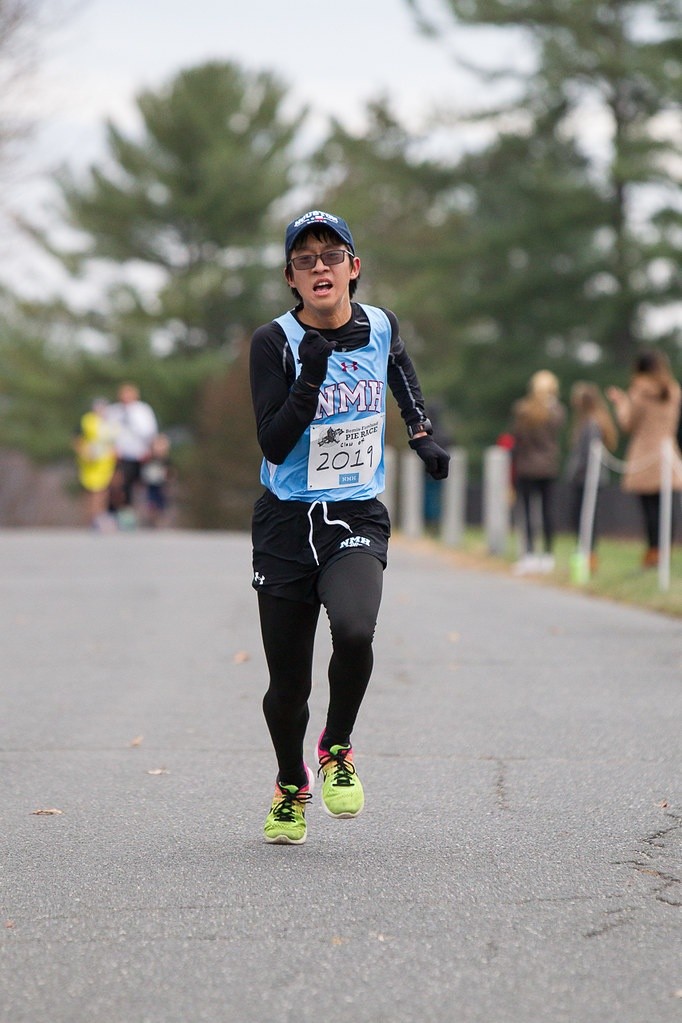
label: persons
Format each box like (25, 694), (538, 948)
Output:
(73, 380), (172, 531)
(569, 380), (618, 571)
(507, 369), (567, 555)
(601, 349), (681, 571)
(250, 210), (451, 842)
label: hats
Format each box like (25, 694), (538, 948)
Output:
(284, 210), (356, 254)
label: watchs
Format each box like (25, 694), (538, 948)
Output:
(407, 417), (433, 439)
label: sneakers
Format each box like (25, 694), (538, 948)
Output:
(317, 728), (365, 820)
(262, 763), (314, 846)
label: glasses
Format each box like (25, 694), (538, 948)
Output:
(286, 250), (355, 270)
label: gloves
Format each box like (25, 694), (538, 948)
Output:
(408, 435), (450, 481)
(297, 330), (337, 384)
